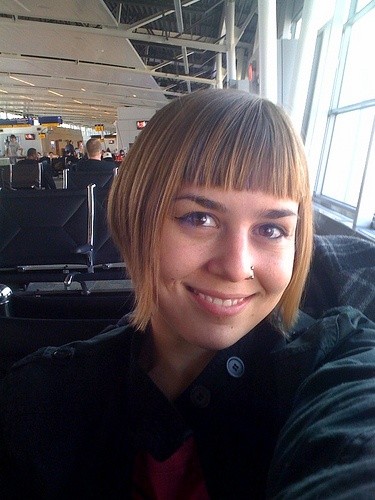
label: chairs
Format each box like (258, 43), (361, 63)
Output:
(0, 156), (135, 375)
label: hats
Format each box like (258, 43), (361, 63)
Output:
(10, 134), (16, 138)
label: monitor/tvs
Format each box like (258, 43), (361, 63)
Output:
(25, 134), (35, 140)
(137, 121), (148, 129)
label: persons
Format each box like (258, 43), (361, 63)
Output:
(65, 139), (85, 164)
(116, 150), (127, 160)
(0, 89), (375, 500)
(47, 152), (57, 176)
(69, 139), (117, 171)
(3, 135), (22, 163)
(16, 148), (57, 190)
